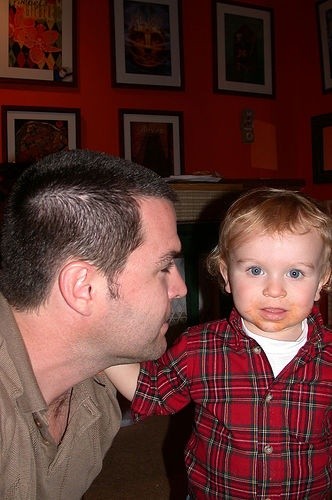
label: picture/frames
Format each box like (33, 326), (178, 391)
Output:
(1, 0), (80, 93)
(118, 108), (185, 178)
(315, 0), (332, 95)
(109, 0), (186, 93)
(310, 112), (332, 184)
(2, 105), (81, 165)
(210, 0), (277, 100)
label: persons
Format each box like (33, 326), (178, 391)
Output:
(135, 130), (175, 178)
(25, 19), (54, 59)
(103, 187), (332, 500)
(0, 149), (188, 500)
(232, 31), (248, 80)
